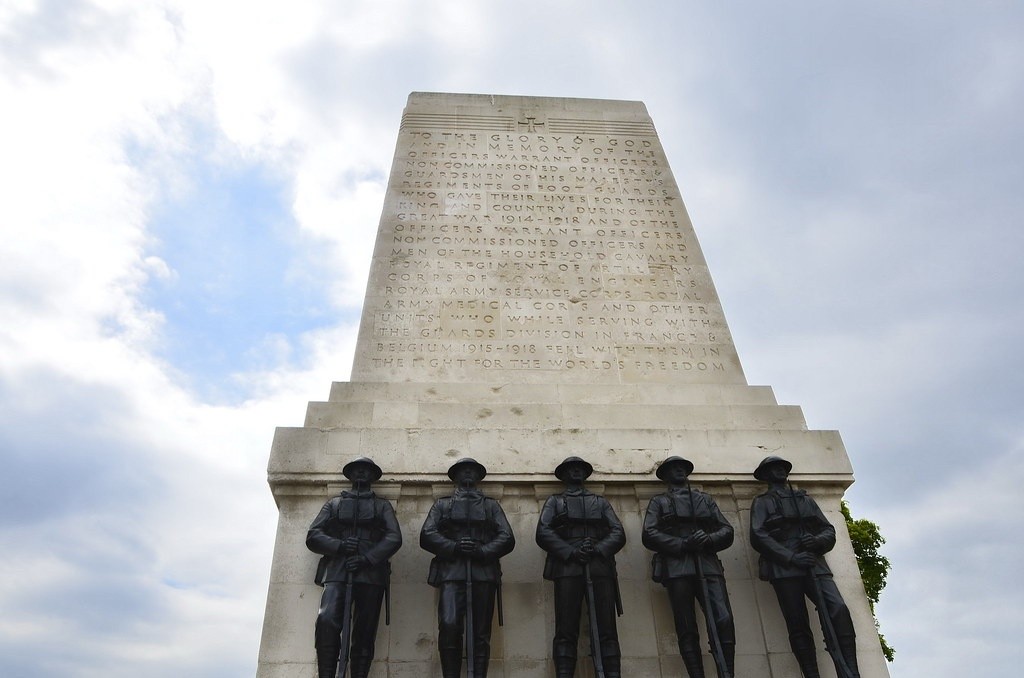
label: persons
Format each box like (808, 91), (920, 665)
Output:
(419, 457), (516, 678)
(305, 456), (402, 678)
(748, 457), (860, 677)
(642, 455), (735, 677)
(535, 457), (626, 678)
